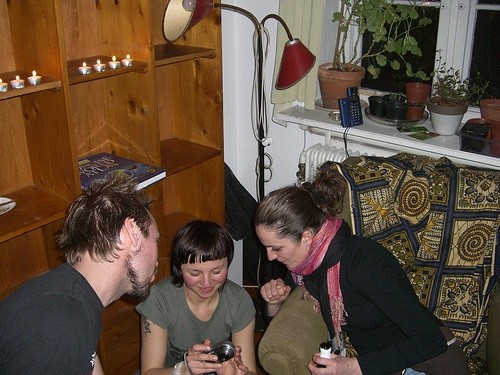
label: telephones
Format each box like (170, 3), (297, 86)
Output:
(339, 87), (363, 128)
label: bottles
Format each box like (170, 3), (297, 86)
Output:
(316, 342), (331, 368)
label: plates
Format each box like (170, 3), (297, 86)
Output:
(0, 196), (16, 215)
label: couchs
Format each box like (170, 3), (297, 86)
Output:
(256, 155), (500, 375)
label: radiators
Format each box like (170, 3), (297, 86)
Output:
(296, 144), (376, 184)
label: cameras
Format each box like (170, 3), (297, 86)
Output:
(203, 340), (236, 364)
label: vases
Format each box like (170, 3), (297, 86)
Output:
(405, 81), (429, 103)
(478, 98), (500, 143)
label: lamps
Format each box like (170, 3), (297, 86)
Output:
(162, 0), (317, 202)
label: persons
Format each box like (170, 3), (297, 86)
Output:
(254, 161), (470, 375)
(0, 177), (160, 375)
(134, 220), (258, 375)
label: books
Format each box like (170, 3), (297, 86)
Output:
(77, 152), (167, 194)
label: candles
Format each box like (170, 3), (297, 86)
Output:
(93, 59), (106, 72)
(10, 75), (24, 89)
(27, 70), (41, 85)
(0, 78), (8, 93)
(78, 62), (91, 75)
(108, 56), (120, 69)
(121, 55), (133, 66)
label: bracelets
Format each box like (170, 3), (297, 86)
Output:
(173, 361), (185, 375)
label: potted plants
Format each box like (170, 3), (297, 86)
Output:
(368, 93), (425, 121)
(427, 48), (489, 135)
(318, 0), (434, 110)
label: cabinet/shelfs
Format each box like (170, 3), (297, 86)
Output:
(0, 0), (226, 375)
